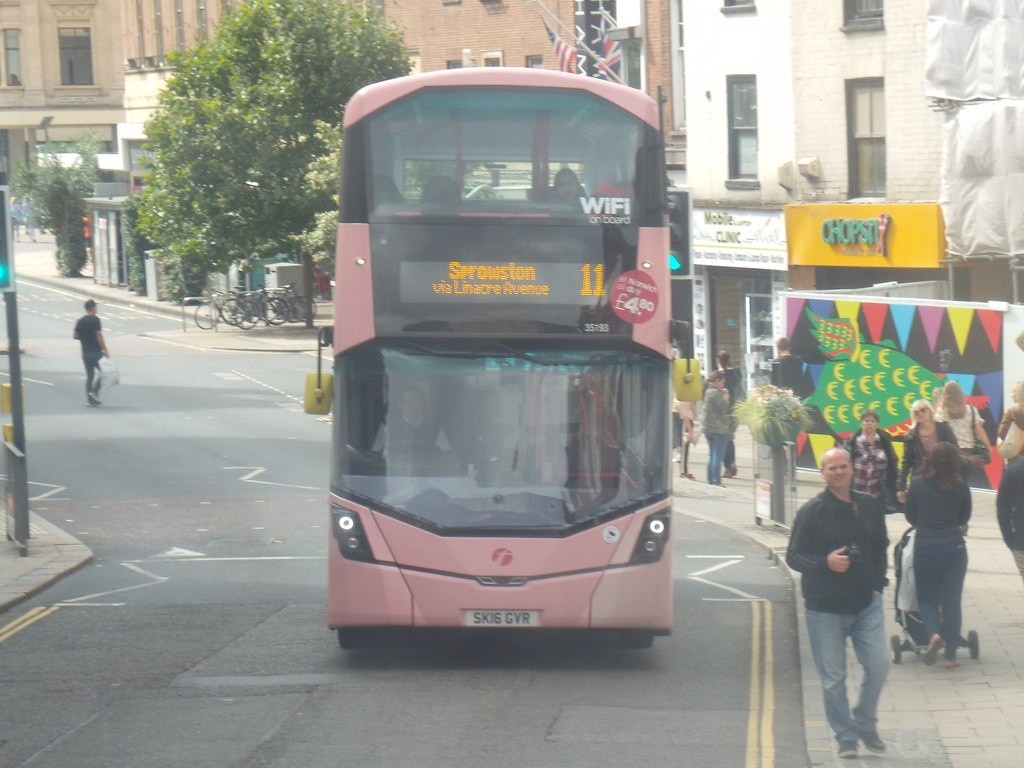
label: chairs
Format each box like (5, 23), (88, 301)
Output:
(421, 174), (462, 203)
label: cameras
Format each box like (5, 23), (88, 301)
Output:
(841, 542), (862, 564)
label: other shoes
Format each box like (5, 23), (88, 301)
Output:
(680, 473), (695, 480)
(853, 722), (885, 753)
(944, 659), (961, 668)
(723, 464), (736, 478)
(924, 633), (942, 665)
(839, 740), (860, 757)
(86, 402), (99, 407)
(88, 392), (102, 405)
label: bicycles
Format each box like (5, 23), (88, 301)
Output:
(194, 281), (317, 330)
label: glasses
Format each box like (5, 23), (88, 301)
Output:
(914, 408), (926, 416)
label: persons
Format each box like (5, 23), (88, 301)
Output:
(847, 410), (900, 514)
(714, 351), (744, 477)
(74, 300), (109, 406)
(8, 74), (21, 85)
(897, 399), (960, 504)
(906, 442), (971, 666)
(787, 448), (891, 757)
(554, 169), (587, 206)
(371, 387), (453, 452)
(772, 337), (814, 480)
(700, 371), (729, 490)
(934, 381), (992, 538)
(997, 379), (1024, 585)
(11, 197), (37, 243)
(672, 349), (685, 464)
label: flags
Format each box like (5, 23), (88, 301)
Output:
(540, 15), (621, 83)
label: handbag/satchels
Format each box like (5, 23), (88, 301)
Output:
(995, 408), (1024, 459)
(97, 356), (119, 388)
(974, 438), (990, 466)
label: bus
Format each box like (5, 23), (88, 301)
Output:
(305, 67), (700, 647)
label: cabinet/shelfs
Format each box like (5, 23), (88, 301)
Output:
(744, 291), (771, 392)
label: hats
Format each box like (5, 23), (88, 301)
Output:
(84, 299), (100, 309)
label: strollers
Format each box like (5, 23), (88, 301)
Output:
(891, 526), (979, 663)
